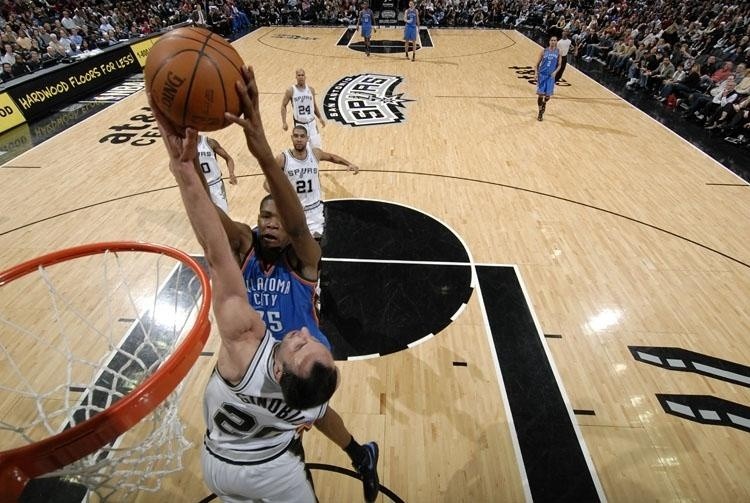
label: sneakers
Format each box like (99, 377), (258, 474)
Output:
(579, 54), (608, 66)
(542, 102), (545, 112)
(365, 46), (367, 51)
(412, 53), (415, 61)
(628, 84), (749, 145)
(352, 440), (380, 503)
(608, 65), (642, 84)
(406, 52), (408, 57)
(537, 111), (542, 120)
(367, 50), (370, 56)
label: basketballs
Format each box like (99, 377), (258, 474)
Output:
(144, 28), (247, 131)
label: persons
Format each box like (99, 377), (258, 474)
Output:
(265, 125), (359, 318)
(0, 1), (749, 150)
(136, 60), (384, 503)
(193, 132), (238, 215)
(155, 124), (343, 503)
